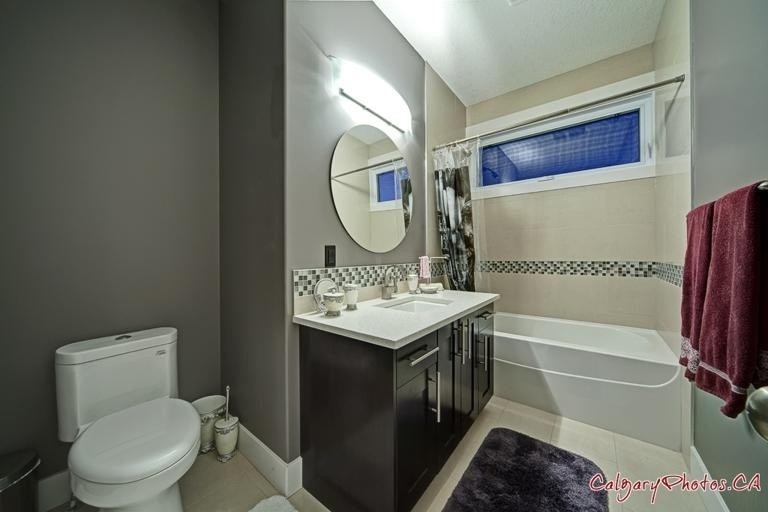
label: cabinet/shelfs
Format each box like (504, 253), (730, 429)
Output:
(474, 302), (495, 418)
(438, 310), (479, 466)
(298, 325), (441, 511)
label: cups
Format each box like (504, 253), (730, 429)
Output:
(343, 283), (358, 309)
(323, 289), (345, 317)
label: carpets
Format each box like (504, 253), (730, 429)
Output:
(439, 426), (607, 512)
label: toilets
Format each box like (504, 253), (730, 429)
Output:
(53, 325), (202, 512)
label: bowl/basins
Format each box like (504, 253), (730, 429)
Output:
(420, 286), (439, 294)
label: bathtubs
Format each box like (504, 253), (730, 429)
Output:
(494, 308), (685, 453)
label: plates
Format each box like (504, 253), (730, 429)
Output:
(313, 278), (340, 310)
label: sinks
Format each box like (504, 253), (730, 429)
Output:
(373, 292), (454, 316)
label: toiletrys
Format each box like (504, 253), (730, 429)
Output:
(407, 265), (419, 293)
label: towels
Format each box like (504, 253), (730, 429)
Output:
(676, 181), (767, 419)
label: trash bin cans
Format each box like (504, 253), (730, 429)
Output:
(0, 448), (43, 512)
(192, 395), (226, 454)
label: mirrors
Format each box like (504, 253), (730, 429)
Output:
(329, 123), (416, 254)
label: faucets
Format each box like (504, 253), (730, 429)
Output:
(382, 266), (395, 299)
